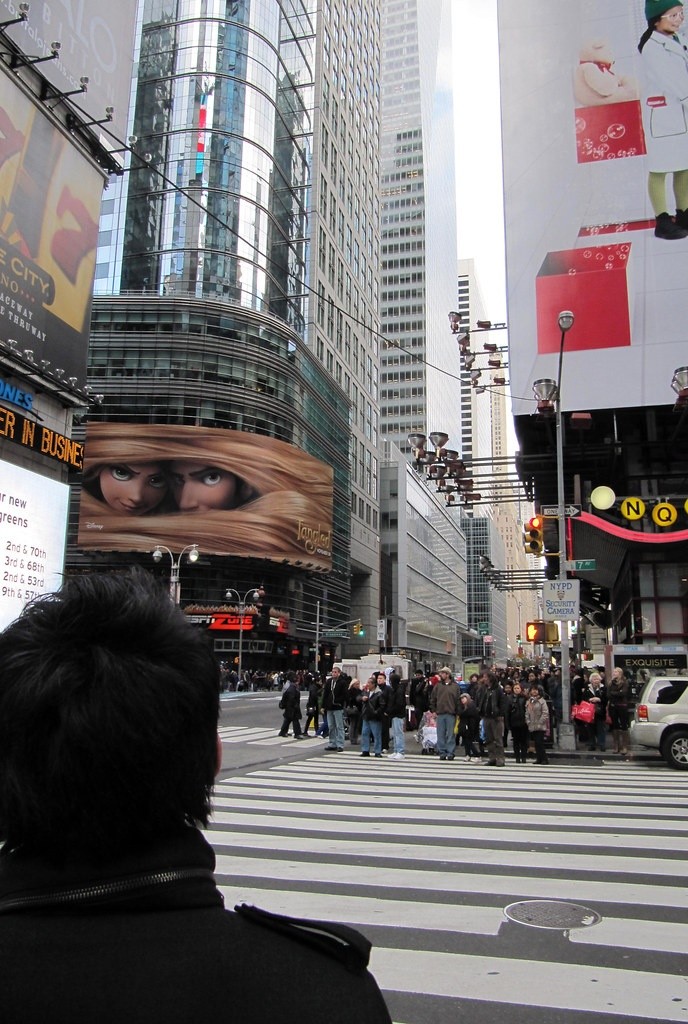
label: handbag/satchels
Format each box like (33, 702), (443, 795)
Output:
(347, 705), (358, 715)
(306, 705), (316, 716)
(605, 706), (612, 724)
(576, 700), (596, 723)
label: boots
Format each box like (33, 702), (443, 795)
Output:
(533, 753), (542, 764)
(542, 753), (549, 765)
(620, 731), (629, 754)
(612, 729), (619, 753)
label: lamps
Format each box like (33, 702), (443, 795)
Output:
(531, 378), (556, 464)
(0, 3), (30, 34)
(449, 312), (510, 393)
(0, 41), (61, 68)
(67, 107), (114, 130)
(41, 77), (89, 111)
(667, 365), (688, 440)
(93, 136), (137, 161)
(480, 553), (548, 591)
(0, 339), (104, 408)
(109, 154), (152, 175)
(409, 432), (536, 507)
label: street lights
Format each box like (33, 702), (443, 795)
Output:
(225, 588), (260, 681)
(152, 544), (199, 608)
(556, 310), (575, 750)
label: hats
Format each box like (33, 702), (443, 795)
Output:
(459, 693), (470, 698)
(438, 667), (451, 674)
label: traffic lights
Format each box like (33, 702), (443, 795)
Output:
(529, 515), (543, 552)
(526, 623), (549, 641)
(523, 522), (534, 553)
(353, 624), (359, 635)
(358, 624), (363, 635)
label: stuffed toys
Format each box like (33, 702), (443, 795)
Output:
(573, 39), (637, 106)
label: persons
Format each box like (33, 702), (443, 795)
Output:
(637, 0), (688, 240)
(218, 658), (322, 693)
(0, 561), (395, 1024)
(276, 662), (653, 766)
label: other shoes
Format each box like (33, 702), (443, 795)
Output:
(588, 746), (595, 751)
(280, 731), (526, 767)
(601, 746), (605, 751)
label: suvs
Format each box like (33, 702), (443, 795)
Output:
(630, 676), (688, 770)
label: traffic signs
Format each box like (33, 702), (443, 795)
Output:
(540, 504), (581, 518)
